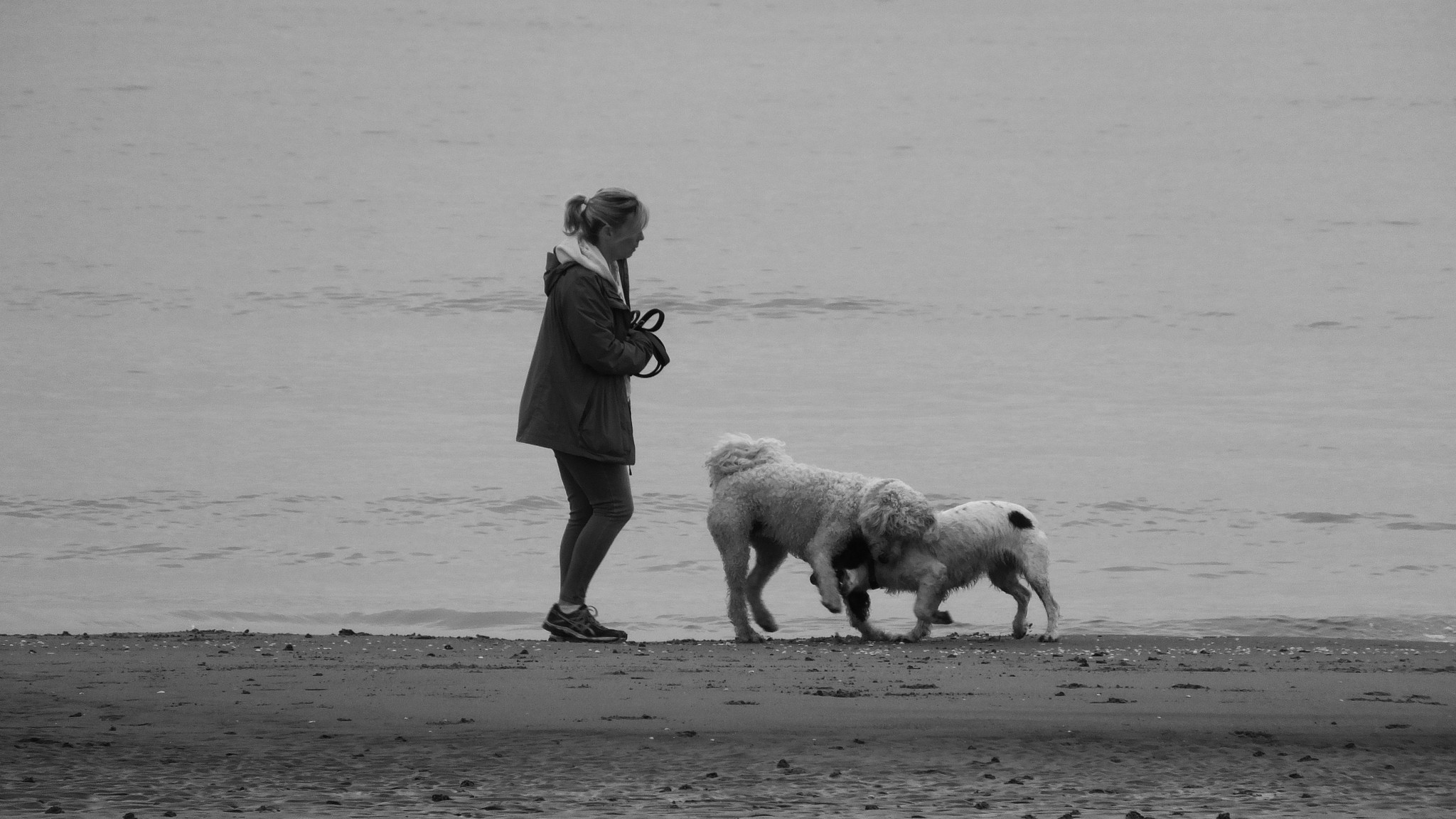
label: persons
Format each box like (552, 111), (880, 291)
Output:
(516, 187), (654, 645)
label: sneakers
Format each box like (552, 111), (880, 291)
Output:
(542, 602), (618, 643)
(549, 629), (627, 642)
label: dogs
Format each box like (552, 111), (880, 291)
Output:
(808, 501), (1061, 644)
(705, 432), (938, 643)
